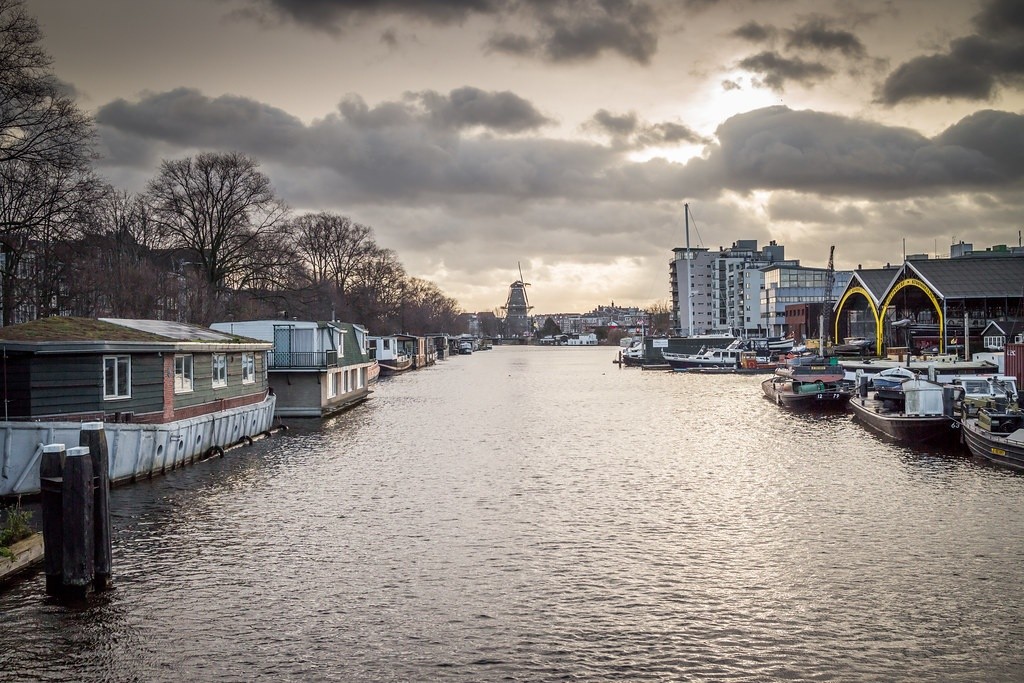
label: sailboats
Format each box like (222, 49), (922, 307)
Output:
(622, 202), (795, 371)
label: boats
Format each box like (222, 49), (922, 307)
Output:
(762, 365), (857, 410)
(367, 332), (413, 376)
(849, 365), (1024, 473)
(458, 343), (472, 355)
